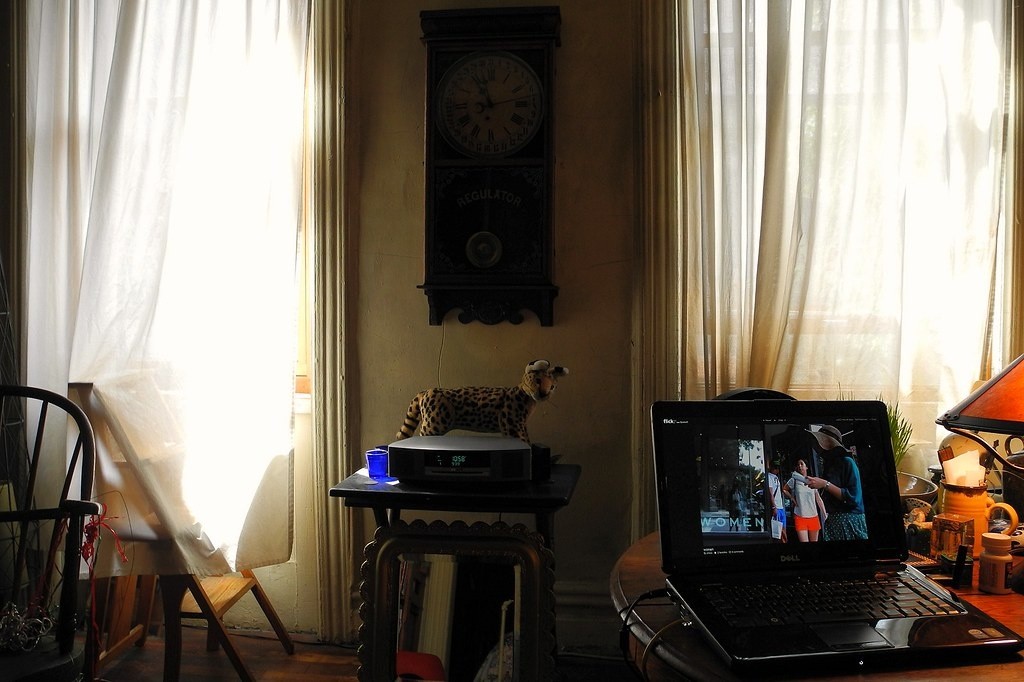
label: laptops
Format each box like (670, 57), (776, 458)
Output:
(650, 400), (1023, 667)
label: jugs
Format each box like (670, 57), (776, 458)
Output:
(938, 480), (1020, 558)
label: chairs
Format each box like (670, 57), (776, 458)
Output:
(0, 385), (95, 681)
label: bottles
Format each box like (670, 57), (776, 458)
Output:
(979, 533), (1013, 595)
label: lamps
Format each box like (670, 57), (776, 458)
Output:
(935, 355), (1024, 589)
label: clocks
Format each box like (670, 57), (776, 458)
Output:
(420, 5), (565, 327)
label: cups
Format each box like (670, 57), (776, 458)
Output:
(366, 449), (388, 477)
(1002, 453), (1024, 522)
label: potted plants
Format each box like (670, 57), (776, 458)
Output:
(825, 383), (939, 509)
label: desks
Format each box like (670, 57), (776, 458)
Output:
(608, 509), (1024, 682)
(329, 459), (583, 681)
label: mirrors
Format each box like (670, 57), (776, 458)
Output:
(354, 519), (556, 680)
